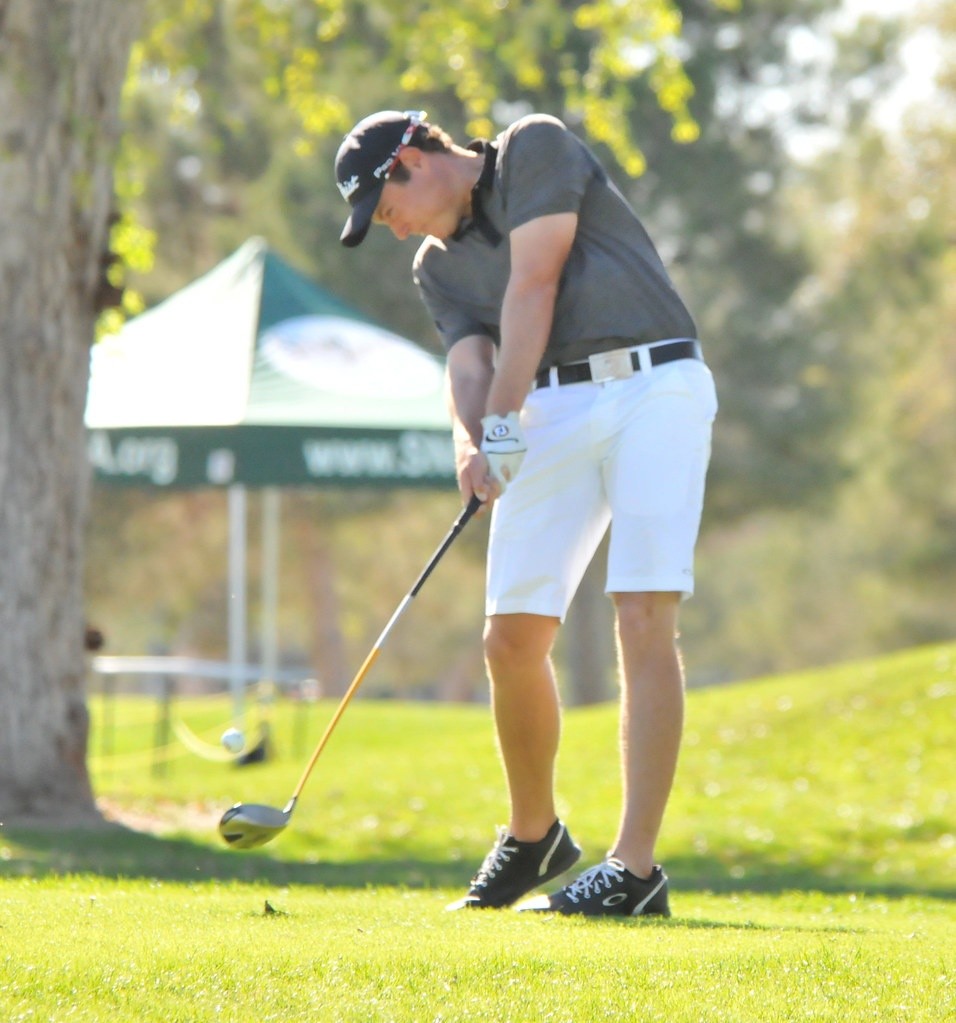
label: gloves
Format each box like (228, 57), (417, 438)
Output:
(478, 411), (525, 491)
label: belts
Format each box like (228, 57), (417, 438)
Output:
(537, 338), (704, 388)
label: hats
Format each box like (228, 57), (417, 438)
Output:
(334, 109), (417, 248)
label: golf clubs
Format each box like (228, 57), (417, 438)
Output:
(220, 492), (482, 849)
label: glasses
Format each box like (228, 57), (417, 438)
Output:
(372, 106), (426, 182)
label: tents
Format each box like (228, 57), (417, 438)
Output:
(83, 237), (458, 723)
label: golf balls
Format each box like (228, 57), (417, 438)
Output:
(222, 730), (244, 754)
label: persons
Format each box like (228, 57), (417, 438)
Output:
(335, 110), (718, 916)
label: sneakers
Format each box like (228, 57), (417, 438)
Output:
(511, 848), (670, 919)
(455, 812), (581, 909)
